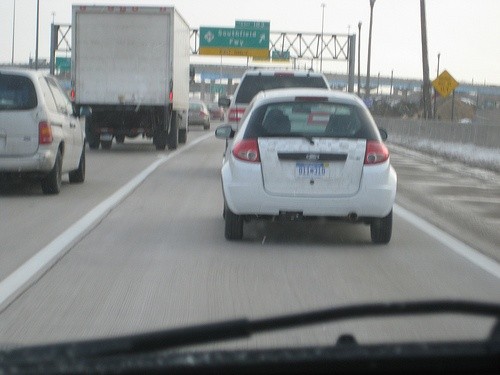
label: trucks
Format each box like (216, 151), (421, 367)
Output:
(70, 4), (192, 151)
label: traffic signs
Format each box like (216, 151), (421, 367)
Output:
(199, 20), (291, 62)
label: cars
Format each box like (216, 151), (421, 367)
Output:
(1, 69), (86, 196)
(214, 88), (398, 245)
(217, 68), (333, 147)
(189, 101), (211, 131)
